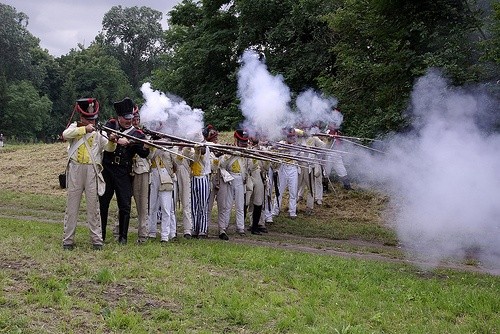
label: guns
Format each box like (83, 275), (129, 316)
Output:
(144, 140), (199, 149)
(279, 153), (327, 161)
(263, 152), (325, 166)
(145, 129), (200, 144)
(248, 151), (280, 162)
(94, 120), (194, 161)
(210, 146), (280, 164)
(257, 131), (351, 167)
(251, 152), (309, 169)
(203, 143), (259, 152)
(308, 133), (383, 143)
(339, 138), (389, 156)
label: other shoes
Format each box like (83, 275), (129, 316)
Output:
(93, 244), (103, 252)
(315, 186), (354, 207)
(115, 233), (208, 248)
(219, 222), (276, 240)
(273, 208), (315, 220)
(63, 244), (72, 253)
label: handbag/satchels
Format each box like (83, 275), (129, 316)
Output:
(158, 167), (174, 192)
(313, 165), (322, 178)
(98, 172), (105, 196)
(245, 172), (255, 192)
(59, 174), (66, 189)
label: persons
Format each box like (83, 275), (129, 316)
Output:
(62, 98), (356, 253)
(0, 133), (4, 147)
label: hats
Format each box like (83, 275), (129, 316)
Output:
(113, 97), (135, 119)
(281, 127), (295, 138)
(76, 98), (99, 120)
(234, 129), (250, 145)
(133, 105), (140, 120)
(203, 124), (218, 140)
